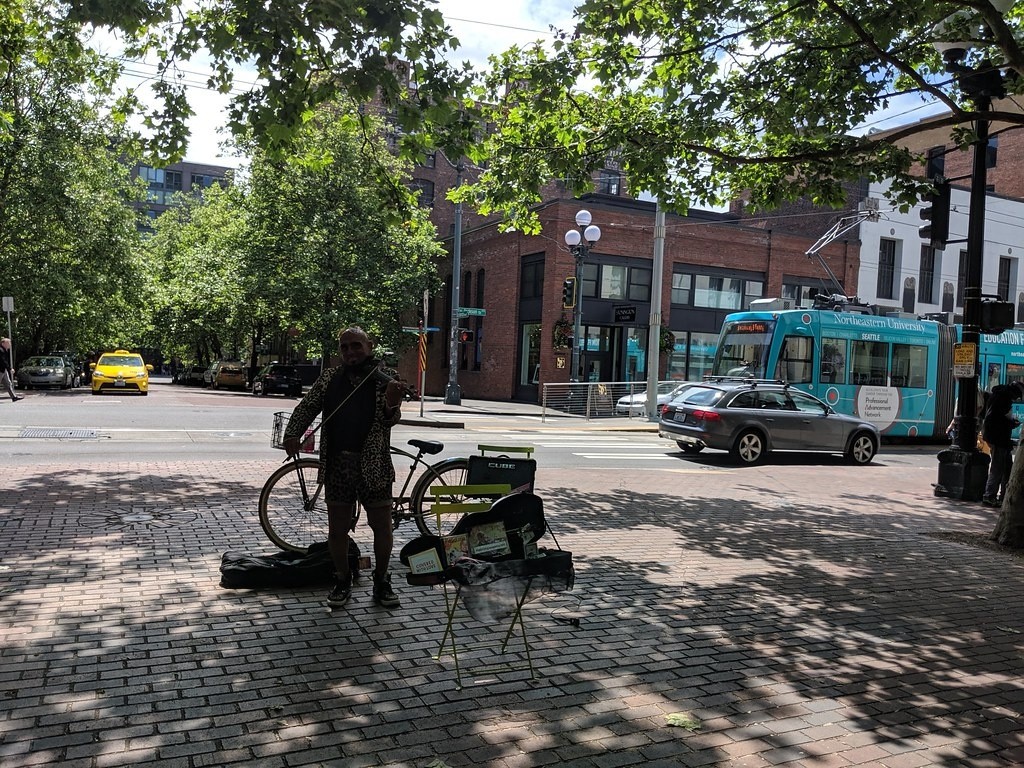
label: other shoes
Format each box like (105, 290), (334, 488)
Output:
(982, 496), (1002, 508)
(12, 397), (23, 402)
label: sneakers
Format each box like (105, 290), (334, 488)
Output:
(371, 570), (400, 606)
(326, 565), (353, 606)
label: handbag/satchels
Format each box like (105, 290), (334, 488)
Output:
(949, 429), (954, 439)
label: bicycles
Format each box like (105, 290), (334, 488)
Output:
(258, 408), (476, 553)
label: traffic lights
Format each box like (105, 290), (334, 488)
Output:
(457, 328), (475, 342)
(919, 184), (949, 251)
(563, 275), (576, 309)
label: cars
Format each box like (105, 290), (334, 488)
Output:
(90, 349), (153, 397)
(172, 362), (244, 389)
(614, 380), (701, 418)
(17, 355), (81, 388)
(250, 361), (303, 397)
(657, 378), (880, 469)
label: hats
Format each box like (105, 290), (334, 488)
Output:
(339, 328), (368, 347)
(1011, 380), (1024, 401)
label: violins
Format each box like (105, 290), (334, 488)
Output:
(349, 360), (422, 402)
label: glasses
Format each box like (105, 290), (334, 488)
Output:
(339, 343), (364, 352)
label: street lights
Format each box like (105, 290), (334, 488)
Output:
(564, 207), (603, 406)
(932, 10), (1008, 499)
(396, 127), (524, 407)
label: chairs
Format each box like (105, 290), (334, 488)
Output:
(429, 482), (538, 691)
(477, 444), (558, 551)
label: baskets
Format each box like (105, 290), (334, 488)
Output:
(270, 411), (322, 455)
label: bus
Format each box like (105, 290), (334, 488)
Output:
(709, 298), (1023, 441)
(569, 336), (719, 412)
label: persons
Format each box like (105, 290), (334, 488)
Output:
(283, 326), (402, 607)
(0, 338), (24, 402)
(931, 375), (1024, 507)
